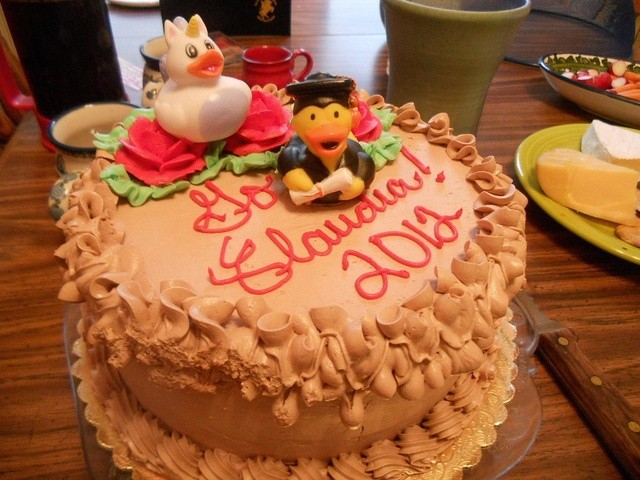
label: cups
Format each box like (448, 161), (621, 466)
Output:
(382, 1), (532, 136)
(241, 45), (313, 91)
(48, 101), (144, 179)
(139, 35), (169, 109)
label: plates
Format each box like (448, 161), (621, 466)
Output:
(514, 123), (640, 265)
(539, 52), (640, 128)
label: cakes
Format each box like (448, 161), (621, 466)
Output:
(52, 82), (530, 479)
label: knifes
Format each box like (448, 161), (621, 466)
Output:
(514, 288), (640, 479)
